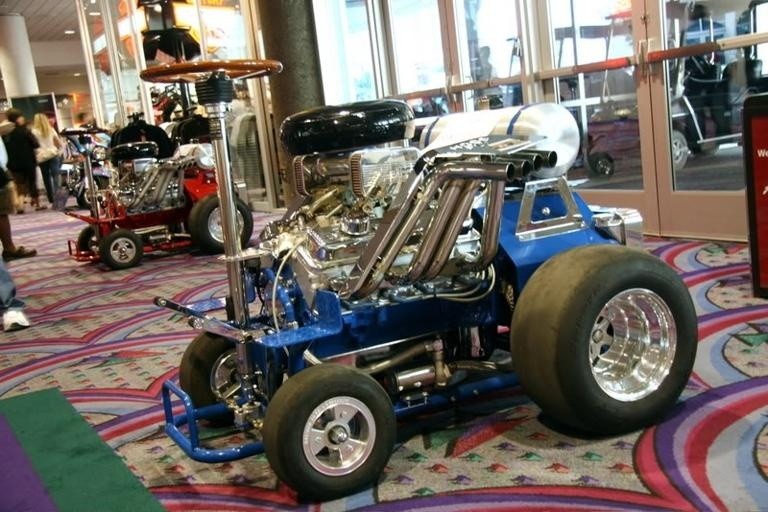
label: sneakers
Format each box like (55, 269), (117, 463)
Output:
(1, 245), (37, 263)
(1, 311), (31, 332)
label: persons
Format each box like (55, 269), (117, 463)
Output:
(0, 133), (38, 260)
(0, 251), (30, 332)
(683, 4), (733, 155)
(2, 108), (46, 214)
(30, 112), (63, 209)
